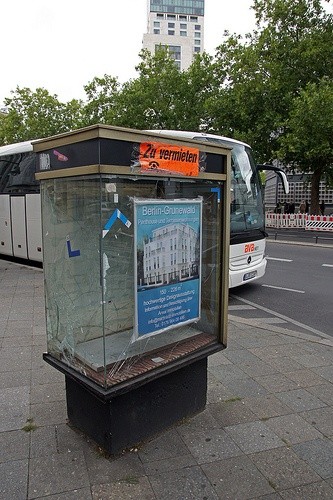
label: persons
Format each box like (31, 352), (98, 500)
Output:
(274, 200), (326, 216)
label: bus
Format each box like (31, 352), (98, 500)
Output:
(0, 129), (291, 291)
